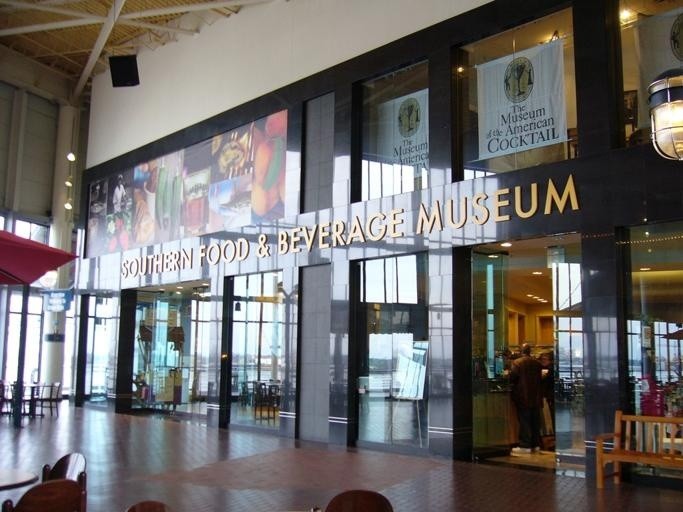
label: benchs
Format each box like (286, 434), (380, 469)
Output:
(593, 409), (681, 491)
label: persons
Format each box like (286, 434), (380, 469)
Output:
(476, 341), (556, 456)
(107, 174), (129, 253)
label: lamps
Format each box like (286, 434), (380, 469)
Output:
(63, 145), (76, 212)
(643, 64), (683, 162)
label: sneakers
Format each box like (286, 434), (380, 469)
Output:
(511, 446), (531, 454)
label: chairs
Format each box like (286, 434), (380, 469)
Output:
(3, 479), (86, 512)
(0, 378), (61, 427)
(239, 377), (295, 427)
(126, 499), (171, 512)
(312, 490), (393, 512)
(43, 452), (87, 486)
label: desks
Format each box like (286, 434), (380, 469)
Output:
(1, 465), (38, 489)
